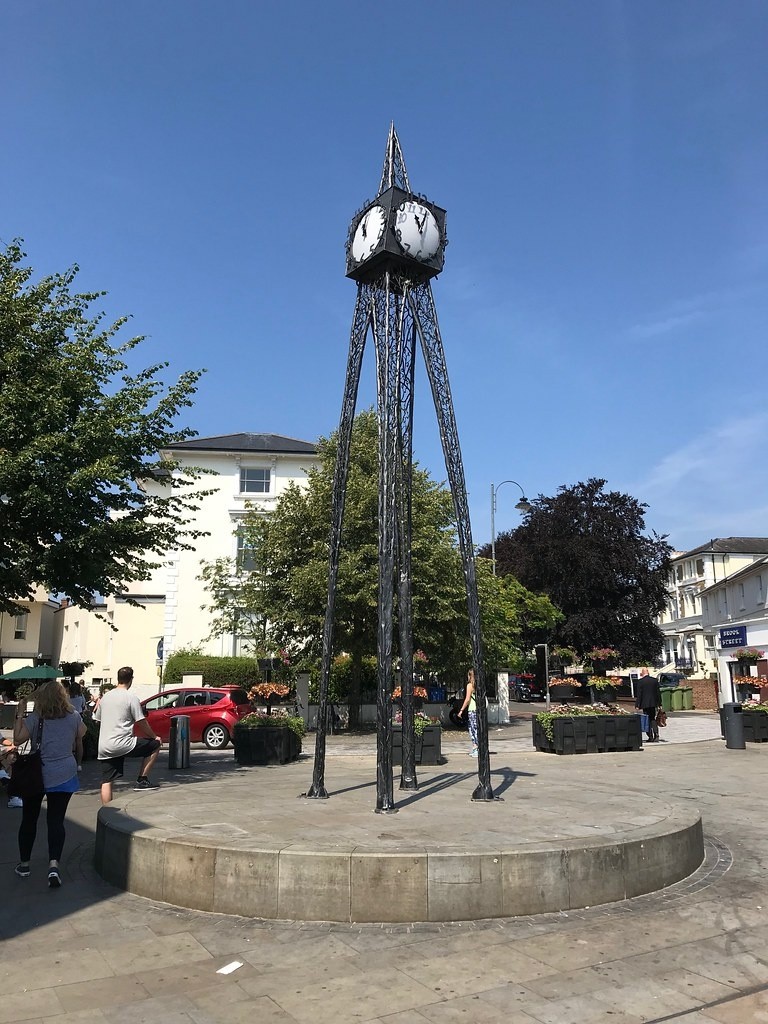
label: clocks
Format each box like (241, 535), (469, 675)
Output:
(388, 189), (447, 262)
(344, 195), (388, 267)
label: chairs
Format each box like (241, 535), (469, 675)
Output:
(185, 696), (194, 706)
(195, 695), (202, 705)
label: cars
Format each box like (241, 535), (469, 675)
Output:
(132, 683), (252, 749)
(521, 675), (544, 695)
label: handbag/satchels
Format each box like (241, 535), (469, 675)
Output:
(656, 709), (667, 726)
(9, 749), (44, 795)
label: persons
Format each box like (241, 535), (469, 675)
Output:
(0, 690), (8, 701)
(96, 666), (163, 805)
(79, 680), (85, 686)
(457, 669), (489, 758)
(69, 683), (86, 770)
(635, 668), (664, 741)
(12, 682), (87, 887)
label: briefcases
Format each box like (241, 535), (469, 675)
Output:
(635, 711), (650, 732)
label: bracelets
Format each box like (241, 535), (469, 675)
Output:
(16, 715), (23, 719)
(460, 710), (464, 712)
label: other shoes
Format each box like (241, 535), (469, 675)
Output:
(646, 735), (659, 742)
(469, 750), (479, 757)
(77, 765), (82, 772)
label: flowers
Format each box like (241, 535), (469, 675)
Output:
(730, 646), (765, 658)
(733, 674), (768, 688)
(247, 683), (290, 700)
(391, 708), (440, 736)
(739, 698), (768, 714)
(536, 702), (631, 742)
(584, 645), (620, 659)
(586, 675), (624, 690)
(391, 685), (428, 701)
(392, 649), (429, 673)
(257, 649), (290, 665)
(548, 646), (576, 658)
(234, 710), (305, 753)
(549, 676), (582, 688)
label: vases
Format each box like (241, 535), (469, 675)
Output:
(551, 656), (573, 665)
(234, 724), (297, 764)
(532, 714), (642, 755)
(550, 686), (575, 698)
(257, 658), (281, 671)
(391, 727), (441, 765)
(591, 685), (617, 701)
(719, 708), (768, 742)
(738, 657), (757, 666)
(592, 656), (614, 670)
(738, 684), (760, 693)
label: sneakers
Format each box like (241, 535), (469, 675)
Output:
(8, 797), (23, 808)
(14, 862), (30, 877)
(134, 779), (160, 790)
(48, 867), (61, 887)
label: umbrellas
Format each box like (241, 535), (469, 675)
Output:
(0, 664), (63, 678)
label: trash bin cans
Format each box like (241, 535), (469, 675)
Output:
(659, 686), (694, 711)
(723, 702), (747, 751)
(170, 714), (190, 771)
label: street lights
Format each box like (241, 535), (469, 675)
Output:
(488, 480), (534, 574)
